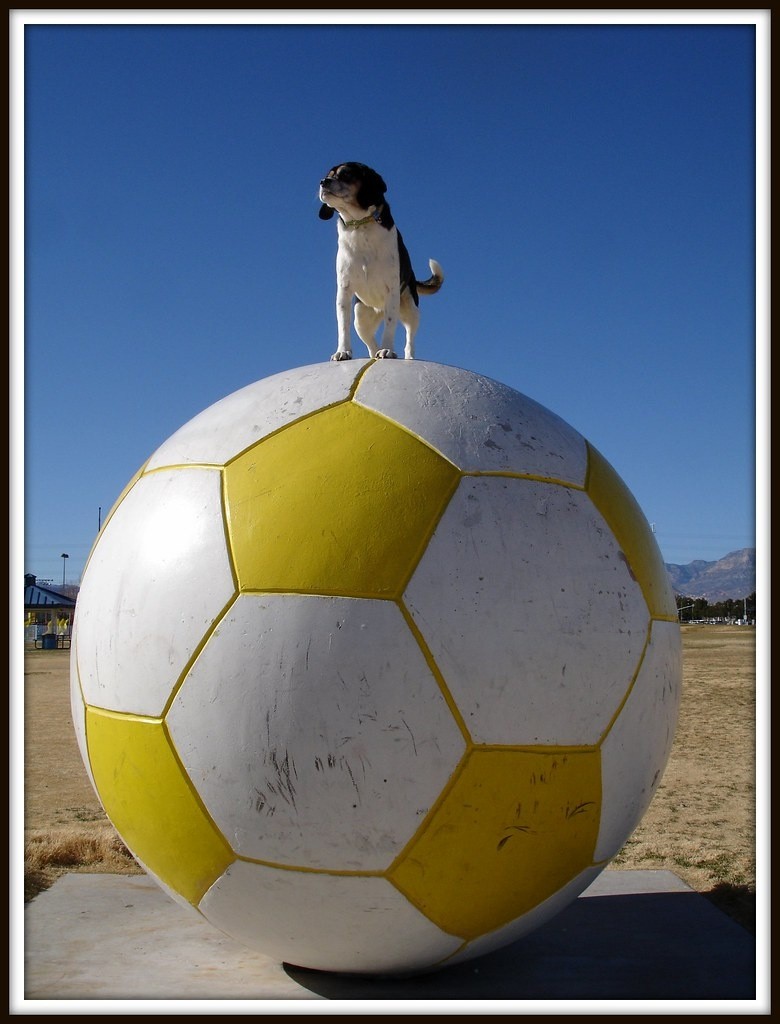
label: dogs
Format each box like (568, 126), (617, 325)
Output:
(318, 162), (444, 361)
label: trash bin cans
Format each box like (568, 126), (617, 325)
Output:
(43, 634), (56, 649)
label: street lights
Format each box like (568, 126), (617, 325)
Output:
(61, 553), (69, 589)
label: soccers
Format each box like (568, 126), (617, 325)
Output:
(64, 350), (688, 980)
